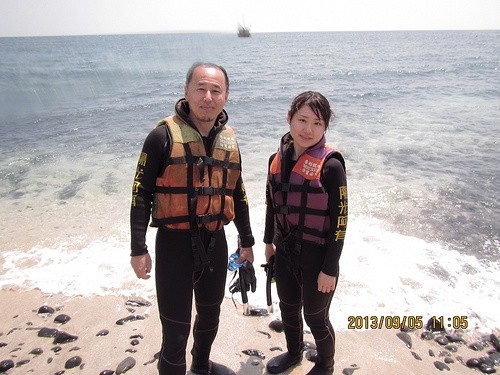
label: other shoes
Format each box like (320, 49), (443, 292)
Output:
(267, 351), (307, 373)
(189, 361), (238, 375)
(305, 364), (334, 375)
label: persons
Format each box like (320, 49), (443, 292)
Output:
(261, 90), (350, 375)
(127, 63), (256, 374)
